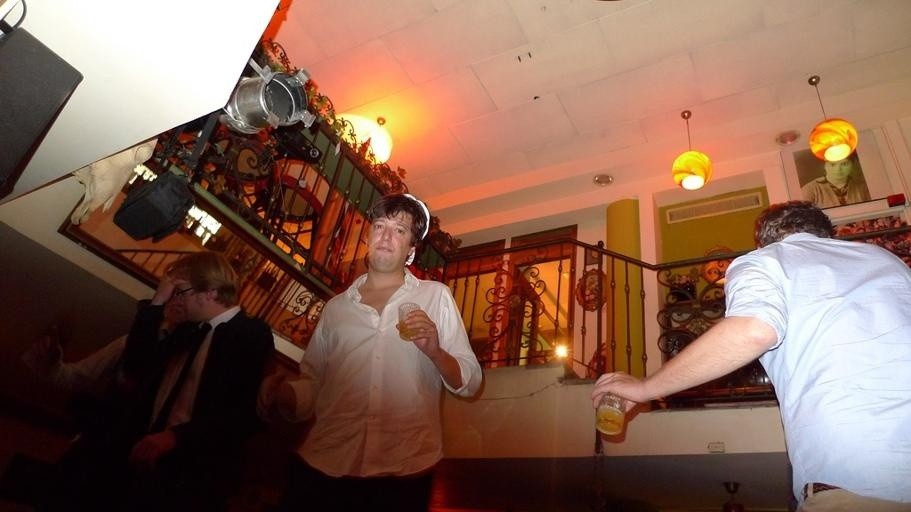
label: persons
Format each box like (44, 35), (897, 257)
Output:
(21, 284), (183, 392)
(62, 249), (275, 511)
(796, 154), (873, 212)
(590, 201), (910, 510)
(252, 191), (485, 512)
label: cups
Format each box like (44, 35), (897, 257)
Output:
(595, 391), (626, 436)
(397, 302), (420, 342)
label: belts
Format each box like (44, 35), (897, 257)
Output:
(803, 483), (838, 499)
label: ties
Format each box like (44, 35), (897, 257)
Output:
(149, 322), (212, 434)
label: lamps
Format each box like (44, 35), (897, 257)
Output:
(671, 110), (713, 192)
(808, 74), (858, 162)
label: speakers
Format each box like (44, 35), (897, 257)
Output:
(0, 27), (84, 199)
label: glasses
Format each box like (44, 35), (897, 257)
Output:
(176, 287), (194, 297)
